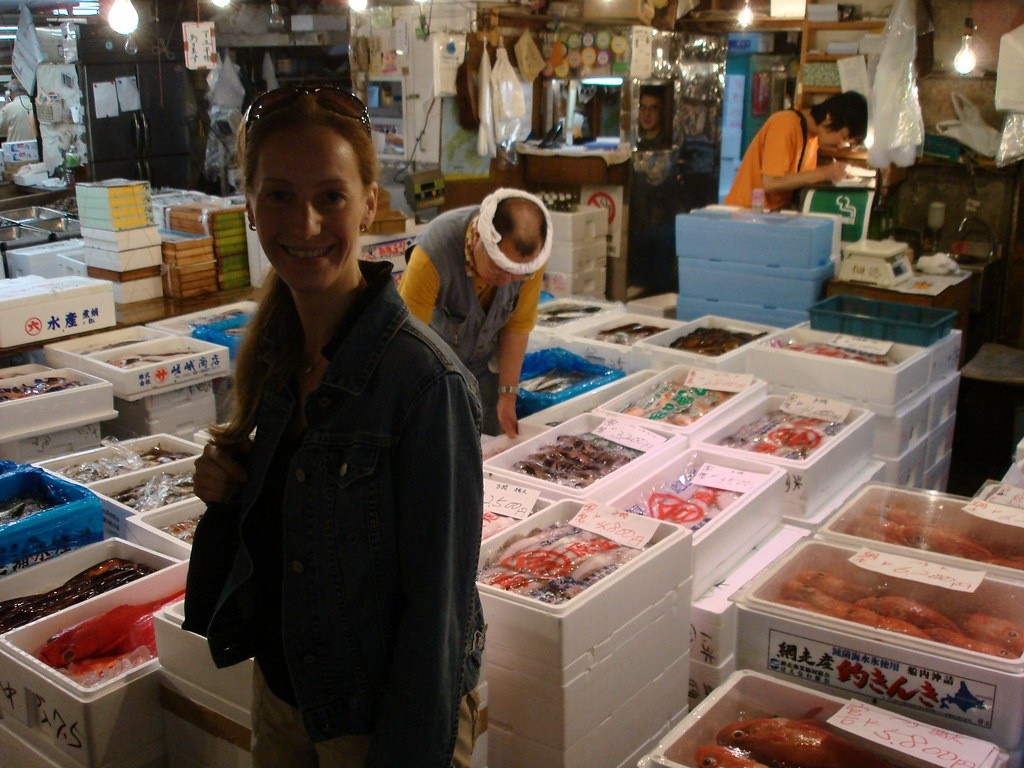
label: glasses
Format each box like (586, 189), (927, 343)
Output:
(245, 81), (373, 166)
(840, 130), (850, 148)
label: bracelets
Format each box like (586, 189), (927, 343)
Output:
(497, 386), (520, 395)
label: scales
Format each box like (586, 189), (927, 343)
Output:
(839, 239), (913, 286)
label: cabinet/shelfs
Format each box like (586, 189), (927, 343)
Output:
(792, 21), (888, 160)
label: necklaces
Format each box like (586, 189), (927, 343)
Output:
(302, 351), (324, 375)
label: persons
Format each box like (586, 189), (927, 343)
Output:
(639, 84), (673, 149)
(723, 90), (869, 212)
(0, 78), (38, 142)
(396, 188), (553, 440)
(191, 87), (484, 768)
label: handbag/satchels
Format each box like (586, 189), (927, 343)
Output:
(181, 499), (241, 637)
(936, 90), (1000, 158)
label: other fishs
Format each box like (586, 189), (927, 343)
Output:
(0, 497), (55, 532)
(838, 497), (1024, 571)
(54, 442), (196, 484)
(109, 470), (197, 512)
(38, 589), (186, 672)
(693, 706), (902, 768)
(0, 558), (160, 633)
(772, 570), (1024, 660)
(106, 351), (206, 370)
(518, 369), (588, 394)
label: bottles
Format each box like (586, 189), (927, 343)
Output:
(65, 145), (81, 169)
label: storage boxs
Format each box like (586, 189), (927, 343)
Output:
(2, 140), (39, 162)
(613, 24), (654, 79)
(14, 173), (48, 185)
(0, 178), (1024, 768)
